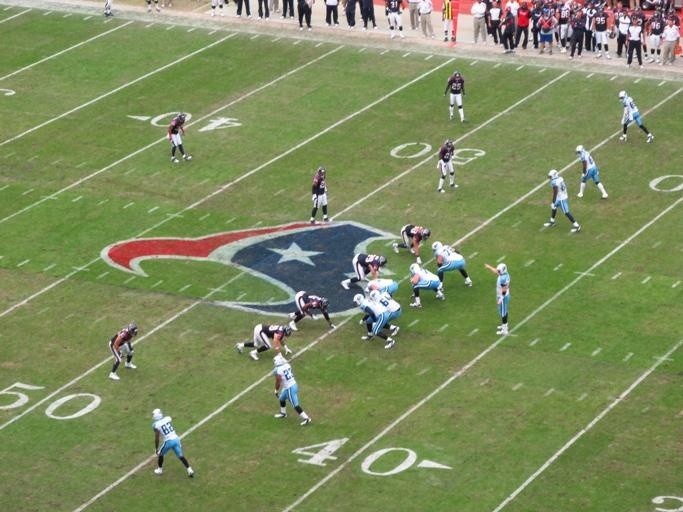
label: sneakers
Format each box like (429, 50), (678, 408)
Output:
(274, 412), (288, 419)
(148, 3), (173, 13)
(325, 16), (456, 42)
(153, 467), (163, 474)
(234, 341), (260, 362)
(125, 362), (137, 369)
(569, 223), (581, 232)
(601, 193), (609, 200)
(103, 12), (112, 17)
(619, 134), (627, 141)
(576, 193), (584, 198)
(288, 311), (299, 332)
(109, 371), (120, 380)
(360, 324), (399, 348)
(310, 216), (316, 223)
(450, 183), (459, 188)
(187, 466), (195, 478)
(496, 322), (510, 336)
(300, 417), (313, 426)
(437, 187), (445, 193)
(464, 279), (472, 287)
(341, 280), (352, 291)
(543, 220), (556, 228)
(473, 37), (569, 55)
(435, 284), (445, 300)
(645, 133), (654, 143)
(569, 49), (683, 67)
(322, 216), (330, 222)
(211, 5), (314, 32)
(171, 157), (180, 163)
(183, 155), (192, 161)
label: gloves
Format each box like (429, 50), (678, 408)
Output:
(358, 319), (364, 325)
(284, 345), (293, 354)
(312, 194), (317, 201)
(118, 351), (124, 358)
(330, 323), (335, 329)
(439, 159), (446, 167)
(170, 139), (177, 147)
(311, 314), (318, 320)
(274, 389), (279, 398)
(128, 348), (135, 356)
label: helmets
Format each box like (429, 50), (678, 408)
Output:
(178, 114), (188, 124)
(319, 296), (329, 310)
(497, 264), (507, 273)
(283, 325), (292, 339)
(619, 90), (629, 101)
(542, 1), (675, 24)
(354, 226), (442, 306)
(128, 323), (138, 336)
(152, 408), (163, 420)
(316, 167), (326, 179)
(549, 169), (558, 179)
(445, 140), (454, 152)
(453, 73), (459, 82)
(577, 143), (586, 154)
(273, 356), (284, 367)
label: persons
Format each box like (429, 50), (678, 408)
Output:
(160, 0), (173, 8)
(617, 90), (654, 144)
(273, 351), (311, 425)
(289, 290), (336, 331)
(167, 113), (192, 164)
(146, 0), (161, 14)
(443, 71), (466, 123)
(236, 322), (293, 360)
(151, 408), (195, 477)
(109, 321), (139, 381)
(310, 167), (329, 225)
(543, 168), (582, 233)
(575, 144), (609, 199)
(441, 1), (456, 42)
(340, 224), (472, 348)
(472, 0), (683, 68)
(210, 0), (437, 40)
(484, 262), (511, 337)
(436, 140), (459, 193)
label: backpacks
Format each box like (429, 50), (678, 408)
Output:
(541, 16), (552, 32)
